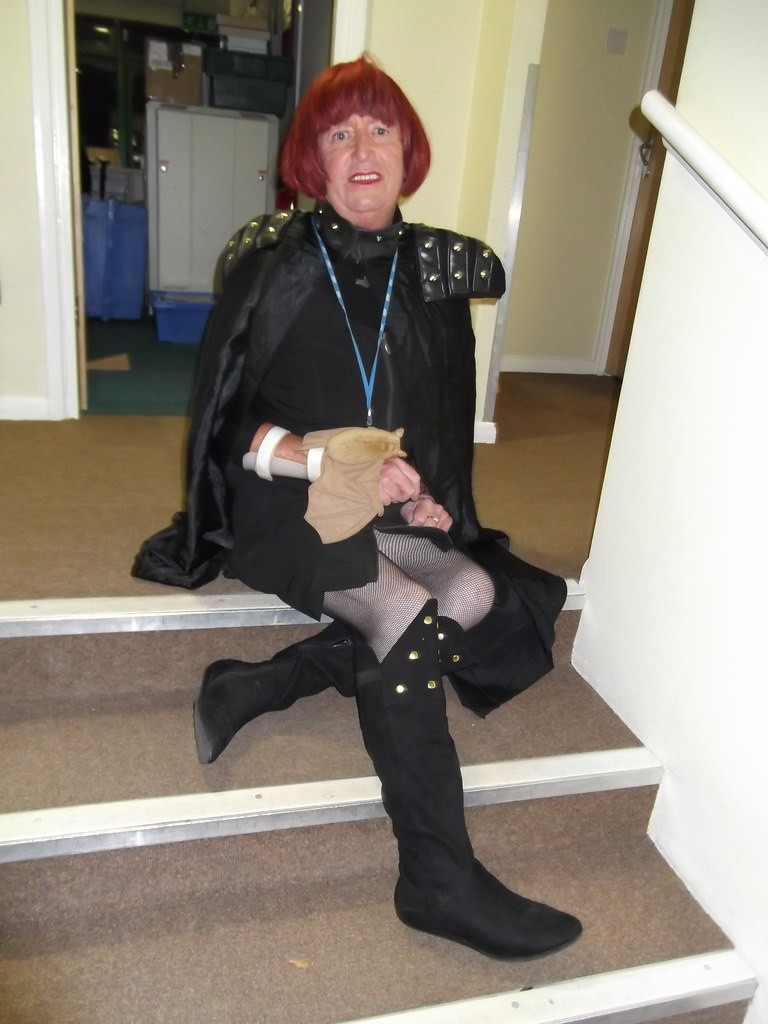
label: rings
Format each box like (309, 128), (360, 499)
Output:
(427, 515), (439, 523)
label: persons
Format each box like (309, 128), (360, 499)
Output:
(133, 61), (581, 962)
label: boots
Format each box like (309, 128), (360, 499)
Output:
(193, 616), (467, 764)
(353, 598), (582, 962)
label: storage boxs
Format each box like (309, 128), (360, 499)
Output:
(143, 38), (204, 107)
(153, 299), (212, 346)
(207, 47), (294, 118)
